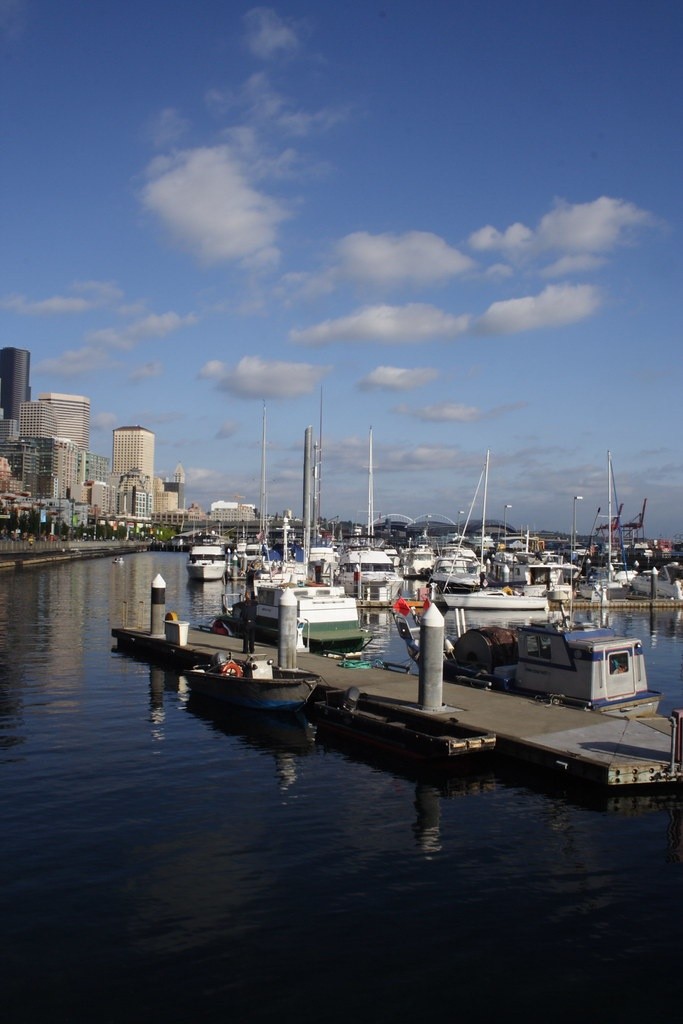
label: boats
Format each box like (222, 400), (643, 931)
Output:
(394, 604), (662, 719)
(213, 576), (375, 657)
(187, 546), (227, 581)
(184, 651), (323, 707)
(399, 447), (683, 599)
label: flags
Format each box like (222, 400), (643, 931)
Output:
(393, 597), (410, 616)
(424, 598), (430, 611)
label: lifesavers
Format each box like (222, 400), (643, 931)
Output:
(309, 580), (326, 587)
(222, 661), (243, 676)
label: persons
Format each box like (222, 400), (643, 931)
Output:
(613, 664), (628, 674)
(244, 564), (257, 601)
(28, 534), (34, 546)
(56, 537), (60, 544)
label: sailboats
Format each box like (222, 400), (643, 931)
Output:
(230, 387), (407, 605)
